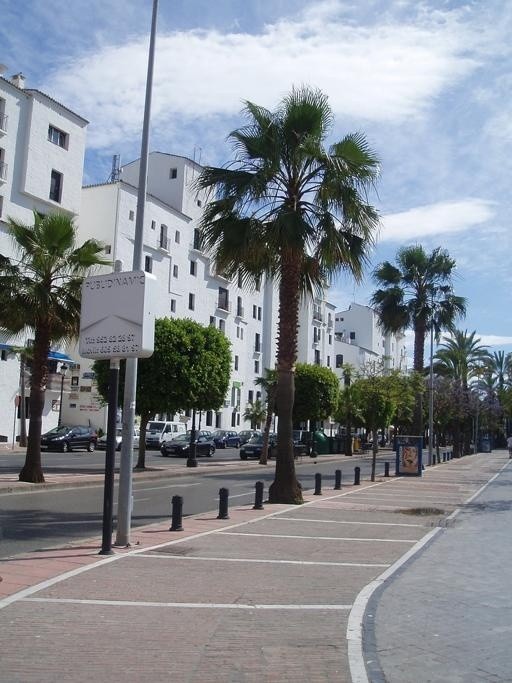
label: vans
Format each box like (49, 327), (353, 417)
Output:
(145, 421), (186, 449)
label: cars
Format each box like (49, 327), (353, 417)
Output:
(40, 424), (98, 453)
(160, 430), (313, 459)
(335, 425), (400, 449)
(96, 427), (148, 451)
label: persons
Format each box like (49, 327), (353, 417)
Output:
(506, 434), (512, 459)
